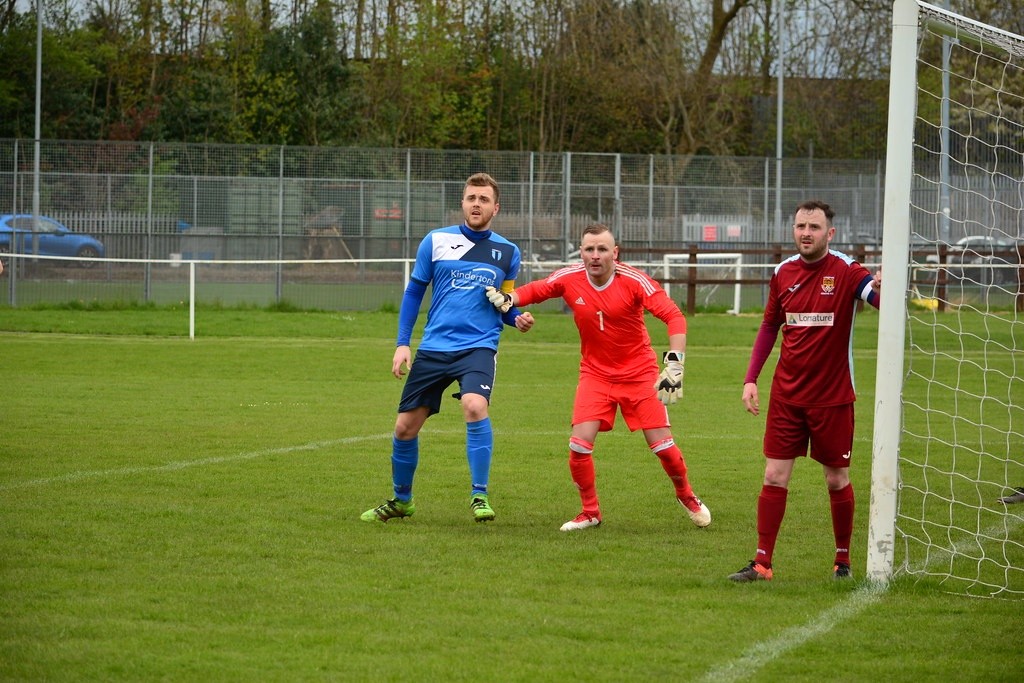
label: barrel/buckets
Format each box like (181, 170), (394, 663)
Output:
(169, 253), (182, 268)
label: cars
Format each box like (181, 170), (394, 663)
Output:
(0, 214), (105, 270)
(842, 230), (1024, 286)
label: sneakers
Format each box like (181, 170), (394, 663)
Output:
(997, 487), (1024, 504)
(675, 491), (712, 528)
(469, 492), (496, 523)
(559, 508), (602, 532)
(727, 560), (773, 582)
(361, 497), (415, 524)
(833, 562), (852, 581)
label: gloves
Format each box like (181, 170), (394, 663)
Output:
(485, 286), (514, 315)
(653, 351), (685, 405)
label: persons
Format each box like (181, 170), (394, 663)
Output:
(360, 171), (533, 525)
(725, 199), (883, 576)
(484, 222), (714, 532)
